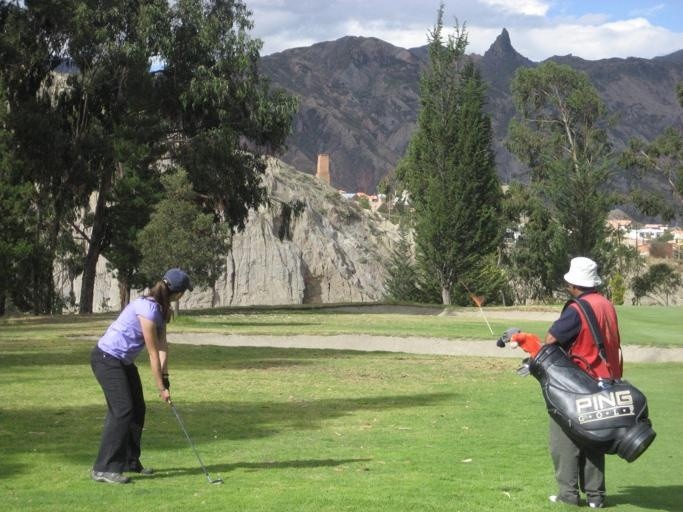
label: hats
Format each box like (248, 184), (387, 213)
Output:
(162, 269), (193, 292)
(563, 256), (602, 288)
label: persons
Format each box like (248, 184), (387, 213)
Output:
(88, 268), (192, 487)
(542, 256), (624, 509)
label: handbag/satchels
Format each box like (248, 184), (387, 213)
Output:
(529, 298), (656, 462)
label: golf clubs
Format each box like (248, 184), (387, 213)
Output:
(166, 398), (222, 485)
(496, 330), (544, 377)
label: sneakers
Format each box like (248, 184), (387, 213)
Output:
(124, 466), (152, 474)
(549, 494), (579, 507)
(587, 500), (605, 508)
(92, 468), (130, 484)
